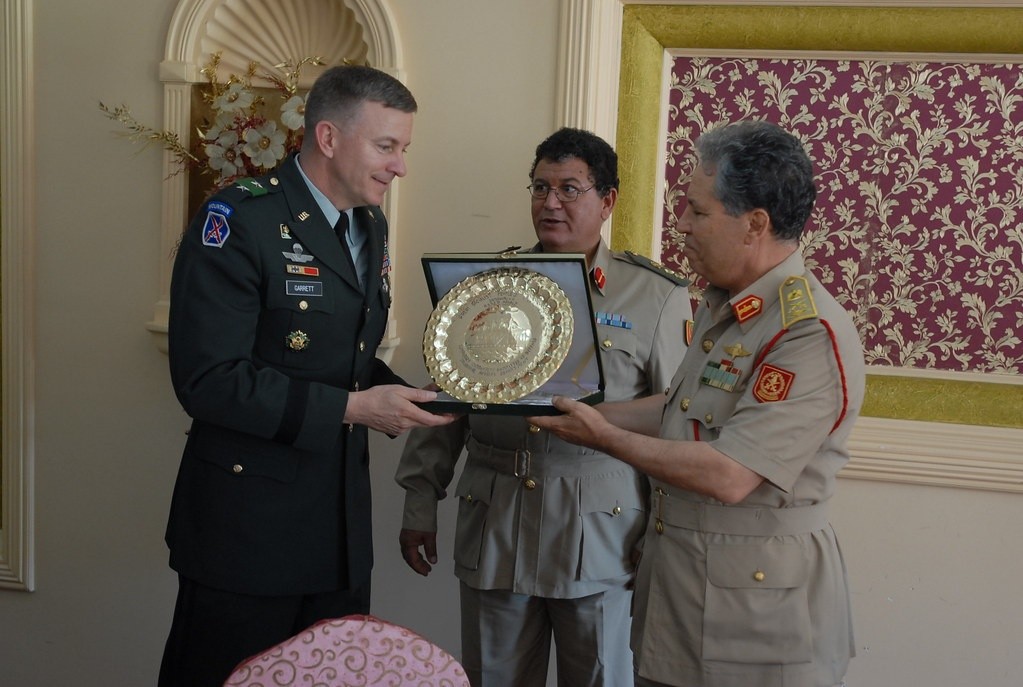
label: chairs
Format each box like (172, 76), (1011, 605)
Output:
(221, 614), (470, 687)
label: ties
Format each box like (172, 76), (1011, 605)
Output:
(334, 210), (360, 290)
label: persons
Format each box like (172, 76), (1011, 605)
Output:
(393, 124), (696, 686)
(153, 63), (463, 685)
(530, 119), (866, 687)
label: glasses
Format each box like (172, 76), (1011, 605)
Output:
(526, 182), (596, 202)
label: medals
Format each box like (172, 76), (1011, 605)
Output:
(421, 266), (575, 403)
